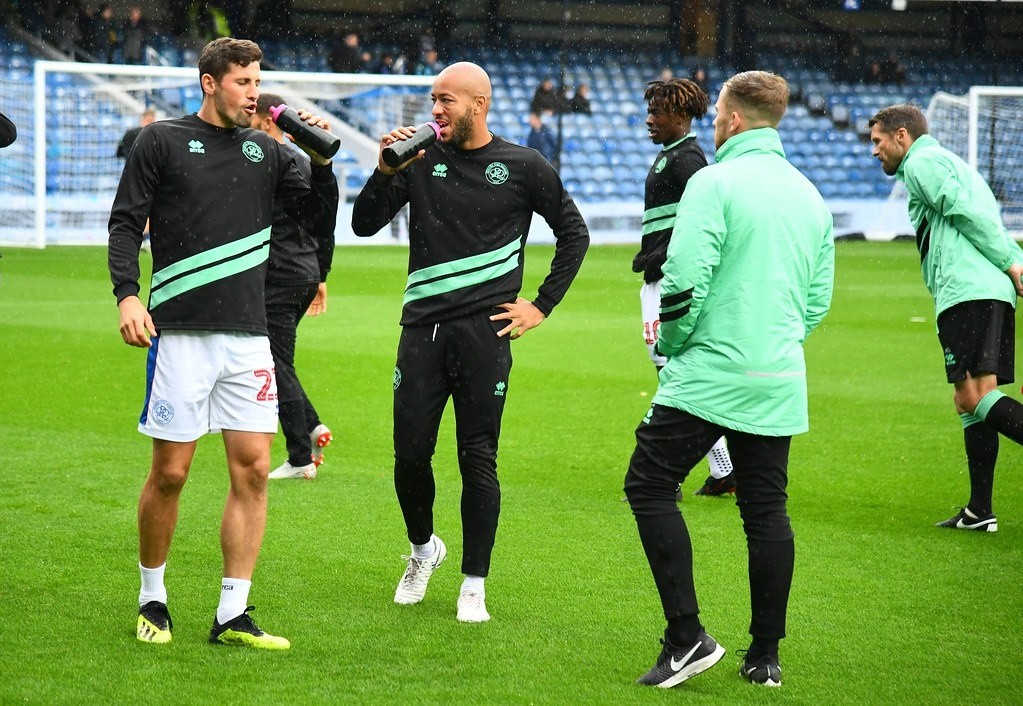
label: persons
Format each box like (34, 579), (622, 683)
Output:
(108, 38), (339, 650)
(251, 94), (334, 481)
(115, 110), (156, 249)
(868, 105), (1023, 532)
(624, 71), (835, 688)
(352, 62), (591, 623)
(835, 43), (905, 84)
(631, 77), (736, 503)
(691, 67), (710, 103)
(17, 0), (147, 63)
(333, 34), (445, 76)
(528, 108), (556, 163)
(532, 80), (591, 117)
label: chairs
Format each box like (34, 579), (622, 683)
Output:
(0, 10), (1023, 232)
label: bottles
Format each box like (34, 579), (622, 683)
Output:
(269, 103), (341, 159)
(382, 122), (441, 168)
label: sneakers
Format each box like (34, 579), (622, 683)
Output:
(638, 625), (727, 690)
(267, 461), (318, 480)
(309, 425), (332, 468)
(456, 583), (490, 622)
(136, 601), (172, 643)
(735, 650), (781, 688)
(394, 535), (447, 605)
(933, 507), (997, 532)
(697, 472), (736, 498)
(209, 605), (291, 651)
